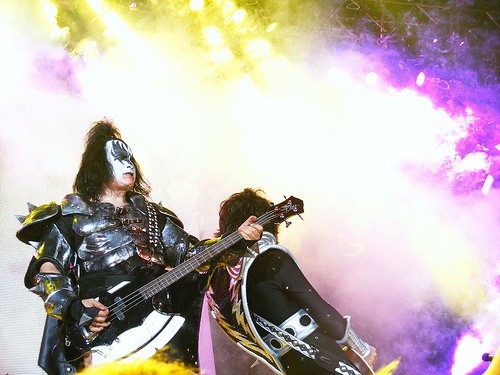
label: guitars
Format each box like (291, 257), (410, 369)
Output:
(56, 196), (304, 371)
(342, 345), (377, 375)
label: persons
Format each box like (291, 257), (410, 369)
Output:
(15, 117), (263, 375)
(206, 188), (378, 375)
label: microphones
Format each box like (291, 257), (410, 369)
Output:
(482, 353), (494, 361)
